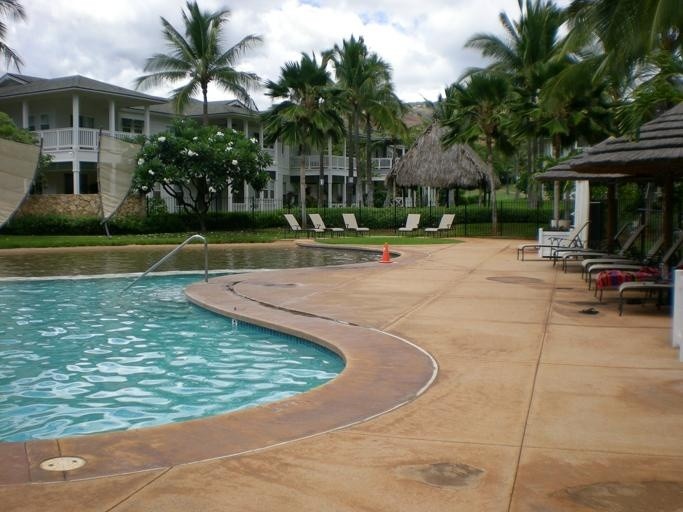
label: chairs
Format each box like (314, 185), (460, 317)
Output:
(516, 220), (682, 317)
(282, 213), (371, 239)
(397, 213), (457, 239)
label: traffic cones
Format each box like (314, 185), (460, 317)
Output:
(379, 241), (392, 263)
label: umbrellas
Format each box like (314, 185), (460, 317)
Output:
(531, 147), (627, 253)
(569, 99), (683, 254)
